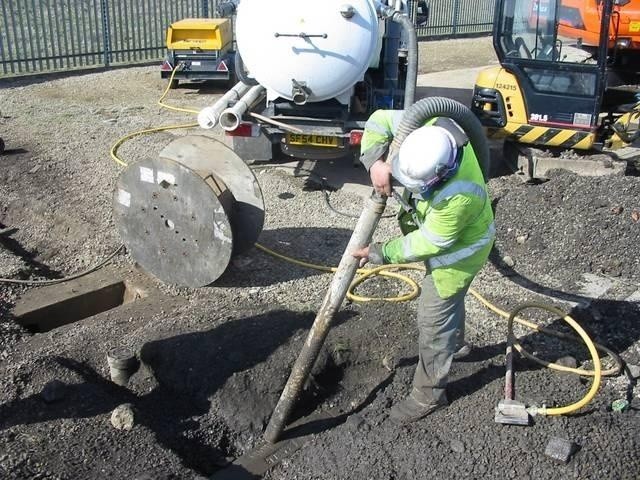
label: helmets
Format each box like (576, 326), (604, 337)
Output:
(391, 126), (459, 195)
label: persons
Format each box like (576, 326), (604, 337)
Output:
(351, 105), (498, 424)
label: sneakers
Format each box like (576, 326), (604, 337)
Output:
(453, 340), (470, 360)
(391, 387), (444, 424)
